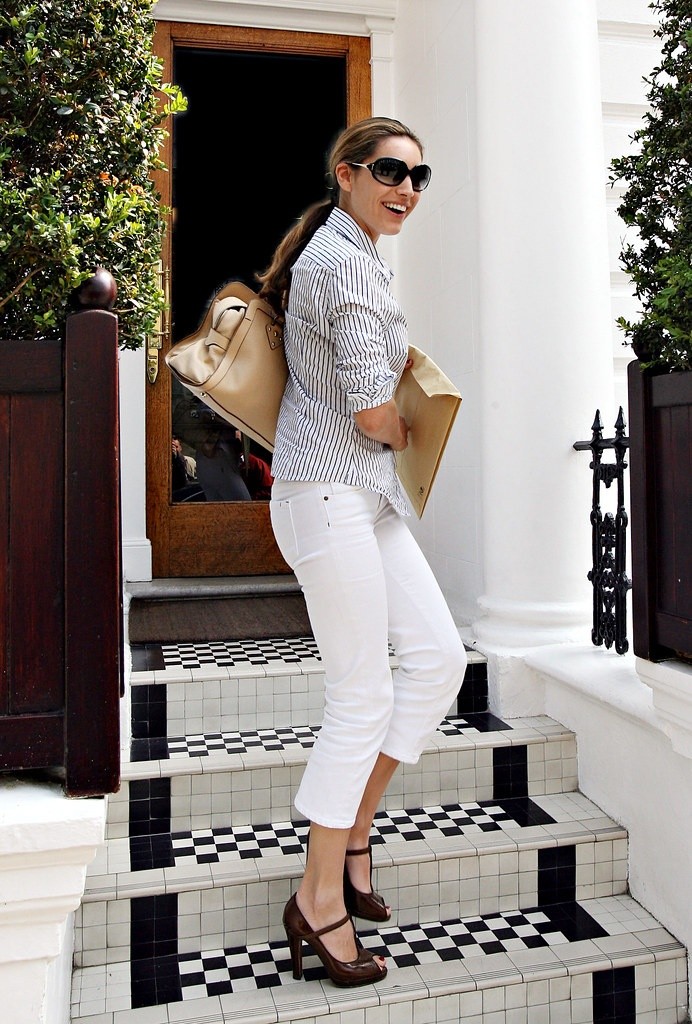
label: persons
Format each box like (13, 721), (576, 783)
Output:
(256, 117), (467, 988)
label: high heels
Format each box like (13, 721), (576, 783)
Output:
(305, 829), (394, 923)
(283, 894), (389, 989)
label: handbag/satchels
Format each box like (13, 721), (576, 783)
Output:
(163, 280), (289, 453)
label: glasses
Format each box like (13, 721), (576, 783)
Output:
(345, 156), (432, 192)
